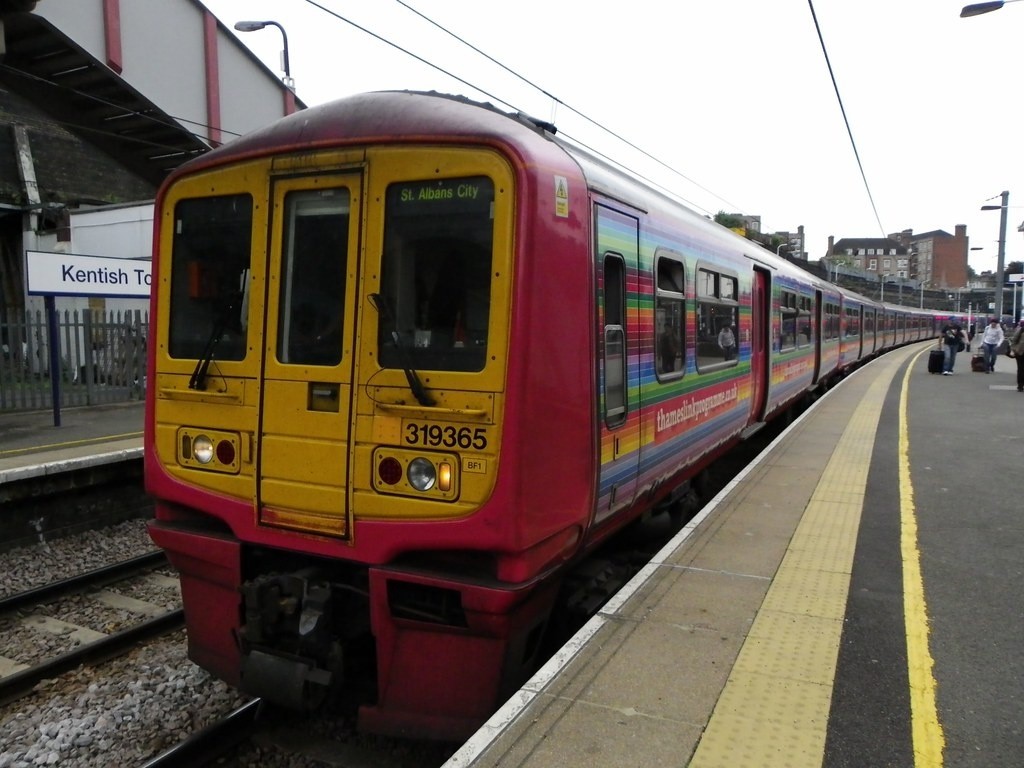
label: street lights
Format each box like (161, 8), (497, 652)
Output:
(234, 20), (290, 78)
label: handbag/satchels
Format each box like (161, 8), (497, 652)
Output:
(958, 342), (965, 352)
(1006, 326), (1024, 358)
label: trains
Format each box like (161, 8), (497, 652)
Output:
(145, 90), (1015, 747)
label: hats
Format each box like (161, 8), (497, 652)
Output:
(948, 315), (957, 321)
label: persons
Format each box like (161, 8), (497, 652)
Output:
(661, 311), (867, 372)
(1012, 325), (1024, 392)
(968, 322), (975, 340)
(938, 315), (970, 376)
(979, 319), (1004, 373)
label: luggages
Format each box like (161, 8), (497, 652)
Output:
(928, 347), (944, 374)
(971, 347), (986, 371)
